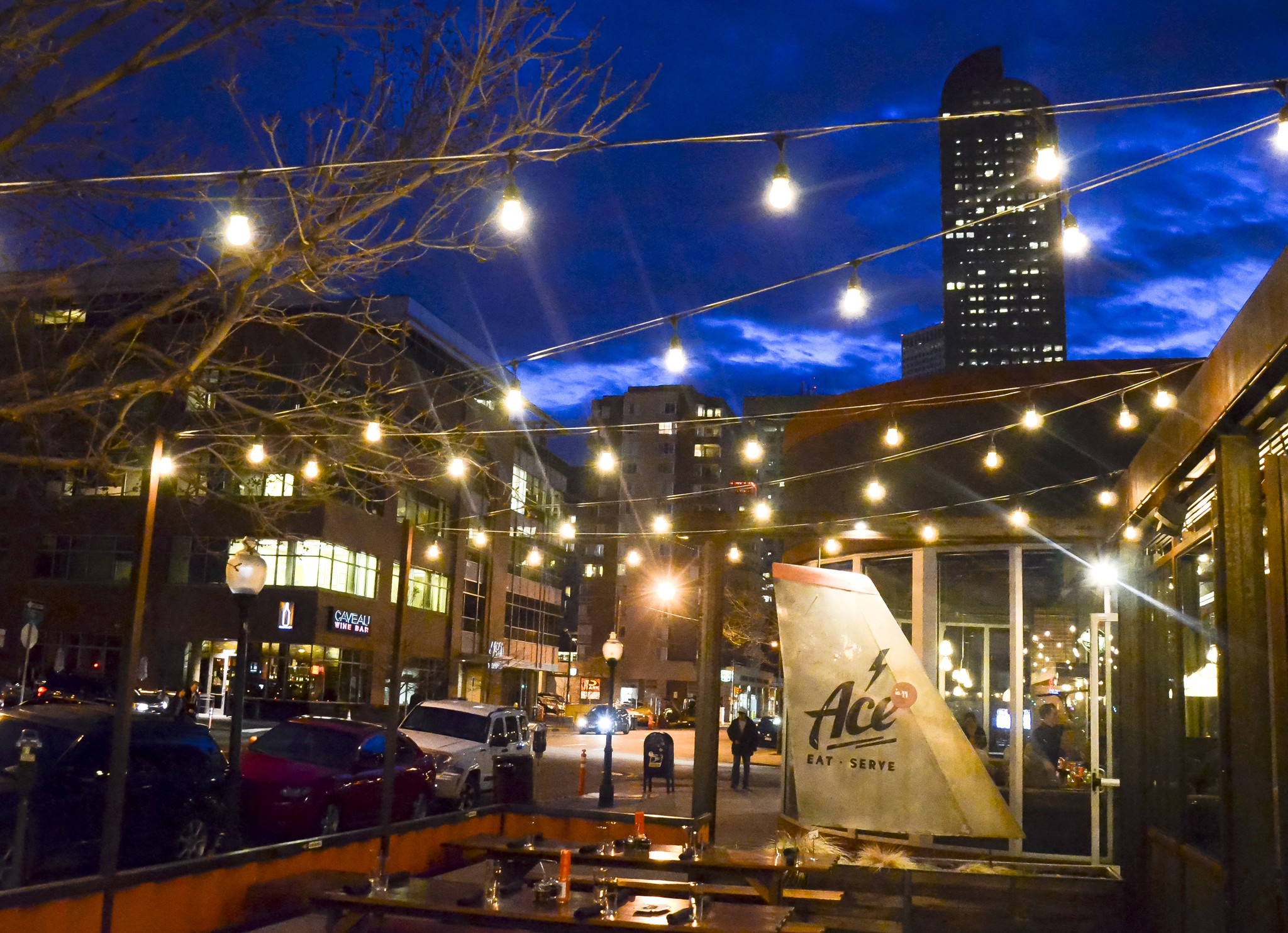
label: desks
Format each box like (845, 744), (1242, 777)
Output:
(255, 833), (843, 933)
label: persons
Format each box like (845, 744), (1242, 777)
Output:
(727, 708), (758, 791)
(998, 735), (1033, 785)
(1031, 703), (1086, 772)
(168, 681), (201, 723)
(959, 712), (986, 749)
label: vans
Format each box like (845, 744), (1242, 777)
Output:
(0, 703), (231, 889)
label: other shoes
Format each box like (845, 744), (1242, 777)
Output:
(741, 784), (747, 788)
(730, 784), (736, 788)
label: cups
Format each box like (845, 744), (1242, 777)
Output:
(485, 859), (501, 912)
(606, 821), (618, 854)
(689, 882), (704, 921)
(593, 868), (619, 921)
(525, 817), (536, 851)
(682, 826), (692, 853)
(795, 838), (806, 865)
(693, 831), (704, 861)
(369, 849), (390, 893)
(596, 826), (607, 854)
(556, 876), (571, 903)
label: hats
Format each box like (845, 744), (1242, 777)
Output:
(738, 707), (747, 713)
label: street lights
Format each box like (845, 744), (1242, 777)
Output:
(597, 630), (624, 808)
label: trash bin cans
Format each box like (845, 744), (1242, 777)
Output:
(491, 754), (535, 805)
(643, 731), (675, 794)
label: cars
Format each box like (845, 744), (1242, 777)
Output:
(396, 697), (533, 808)
(234, 714), (434, 844)
(538, 695), (566, 716)
(754, 716), (781, 748)
(576, 704), (631, 735)
